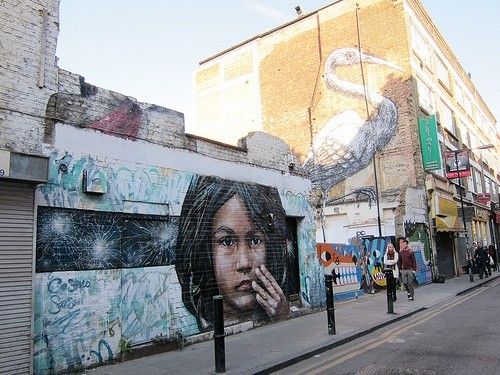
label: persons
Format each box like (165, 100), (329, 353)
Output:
(466, 242), (497, 279)
(384, 244), (399, 301)
(398, 240), (417, 300)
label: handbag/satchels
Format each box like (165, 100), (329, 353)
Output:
(489, 256), (495, 265)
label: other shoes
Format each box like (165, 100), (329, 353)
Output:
(409, 296), (414, 300)
(407, 292), (411, 296)
